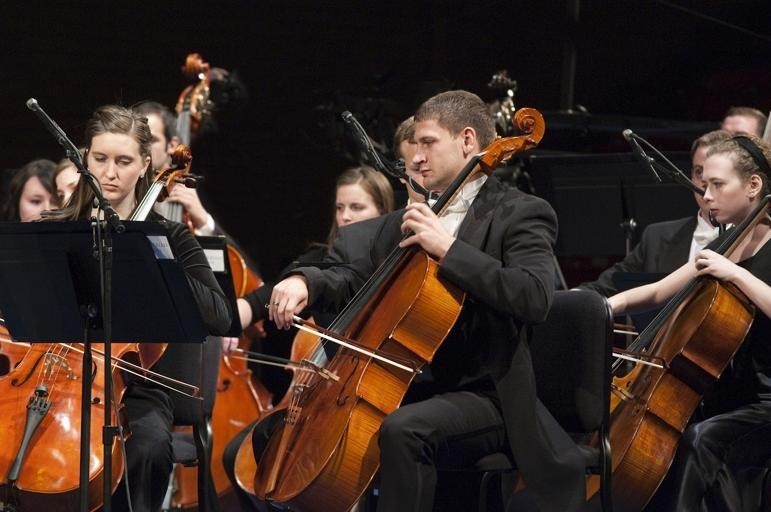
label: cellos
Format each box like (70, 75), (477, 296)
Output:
(233, 106), (545, 512)
(169, 53), (274, 509)
(0, 144), (192, 512)
(586, 196), (771, 512)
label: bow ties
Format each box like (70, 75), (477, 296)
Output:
(427, 196), (467, 216)
(694, 224), (733, 245)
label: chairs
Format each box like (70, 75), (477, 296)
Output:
(467, 291), (614, 511)
(99, 296), (220, 512)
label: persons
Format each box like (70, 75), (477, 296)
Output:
(134, 100), (260, 277)
(0, 160), (57, 222)
(51, 149), (87, 209)
(223, 90), (587, 511)
(607, 131), (771, 512)
(238, 167), (394, 413)
(561, 97), (733, 299)
(41, 106), (230, 512)
(720, 106), (768, 138)
(321, 115), (428, 275)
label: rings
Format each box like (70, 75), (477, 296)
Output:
(174, 189), (177, 192)
(273, 302), (279, 305)
